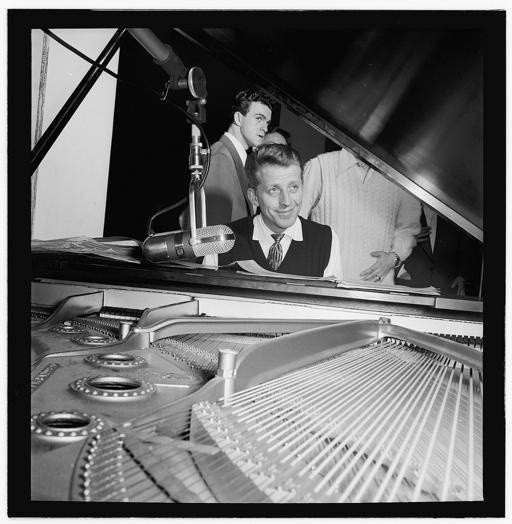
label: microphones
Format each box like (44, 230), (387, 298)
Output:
(140, 222), (236, 264)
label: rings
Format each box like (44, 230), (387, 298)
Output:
(376, 276), (381, 280)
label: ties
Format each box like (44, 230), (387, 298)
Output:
(267, 234), (284, 271)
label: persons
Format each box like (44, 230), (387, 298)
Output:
(262, 126), (291, 145)
(183, 88), (274, 230)
(298, 148), (422, 286)
(394, 202), (467, 297)
(198, 141), (343, 281)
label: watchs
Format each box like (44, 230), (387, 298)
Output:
(392, 252), (401, 267)
